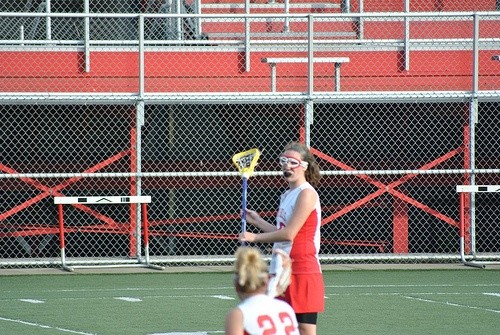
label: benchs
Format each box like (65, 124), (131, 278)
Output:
(261, 55), (351, 92)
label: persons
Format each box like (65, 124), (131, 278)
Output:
(240, 142), (325, 335)
(226, 247), (299, 335)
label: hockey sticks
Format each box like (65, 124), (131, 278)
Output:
(232, 149), (261, 247)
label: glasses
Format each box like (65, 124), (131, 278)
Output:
(279, 156), (309, 169)
